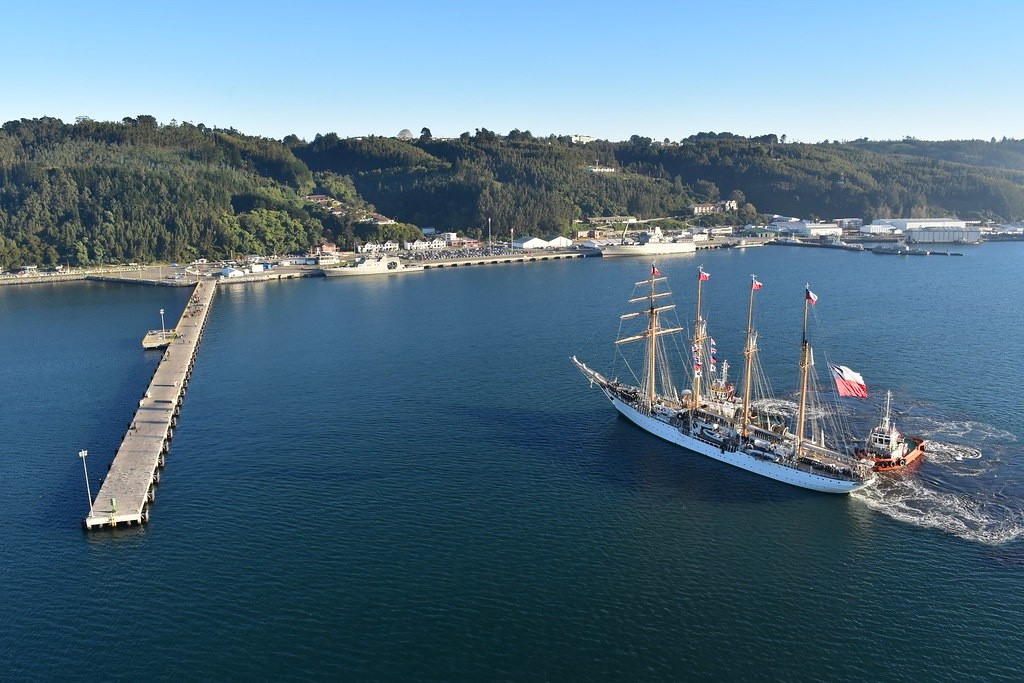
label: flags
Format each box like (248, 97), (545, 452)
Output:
(651, 263), (660, 275)
(692, 344), (703, 377)
(751, 278), (762, 290)
(829, 363), (869, 399)
(806, 288), (819, 305)
(699, 271), (710, 281)
(711, 338), (717, 372)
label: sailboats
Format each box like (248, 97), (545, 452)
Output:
(565, 258), (878, 496)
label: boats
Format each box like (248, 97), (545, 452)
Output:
(853, 388), (927, 472)
(733, 236), (765, 248)
(319, 246), (425, 276)
(595, 218), (697, 257)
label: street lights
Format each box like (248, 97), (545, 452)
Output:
(159, 308), (166, 342)
(78, 449), (95, 518)
